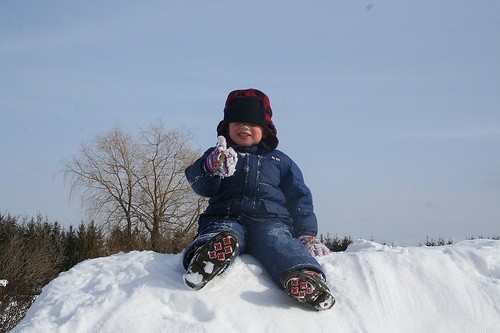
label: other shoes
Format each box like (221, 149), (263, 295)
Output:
(284, 269), (335, 311)
(184, 231), (239, 291)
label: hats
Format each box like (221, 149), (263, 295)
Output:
(217, 89), (279, 154)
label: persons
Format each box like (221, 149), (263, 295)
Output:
(183, 88), (336, 312)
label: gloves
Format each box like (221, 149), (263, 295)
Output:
(206, 136), (238, 179)
(298, 235), (331, 257)
